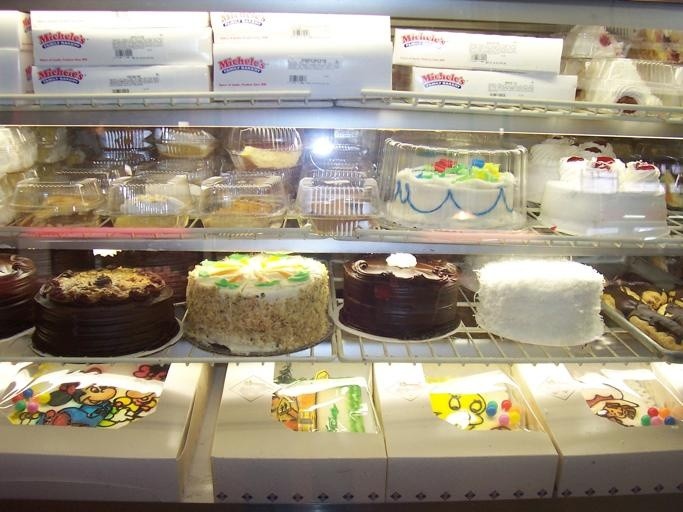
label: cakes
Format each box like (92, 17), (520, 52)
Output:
(464, 255), (572, 290)
(427, 376), (520, 431)
(580, 382), (675, 428)
(0, 253), (39, 340)
(270, 363), (375, 433)
(472, 259), (606, 346)
(528, 135), (683, 236)
(185, 252), (333, 355)
(6, 362), (169, 430)
(338, 252), (461, 341)
(32, 264), (175, 358)
(559, 24), (663, 116)
(387, 158), (515, 227)
(102, 250), (203, 304)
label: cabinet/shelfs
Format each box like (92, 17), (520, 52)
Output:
(0, 0), (683, 511)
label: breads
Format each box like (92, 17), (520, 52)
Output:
(601, 255), (683, 352)
(0, 126), (357, 230)
